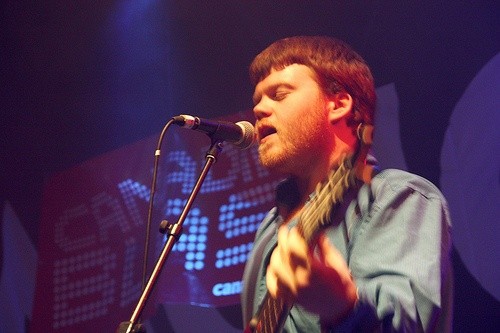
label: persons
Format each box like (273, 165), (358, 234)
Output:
(239, 34), (455, 333)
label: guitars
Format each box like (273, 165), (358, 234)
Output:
(243, 122), (376, 333)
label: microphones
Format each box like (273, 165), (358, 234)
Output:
(173, 113), (254, 150)
